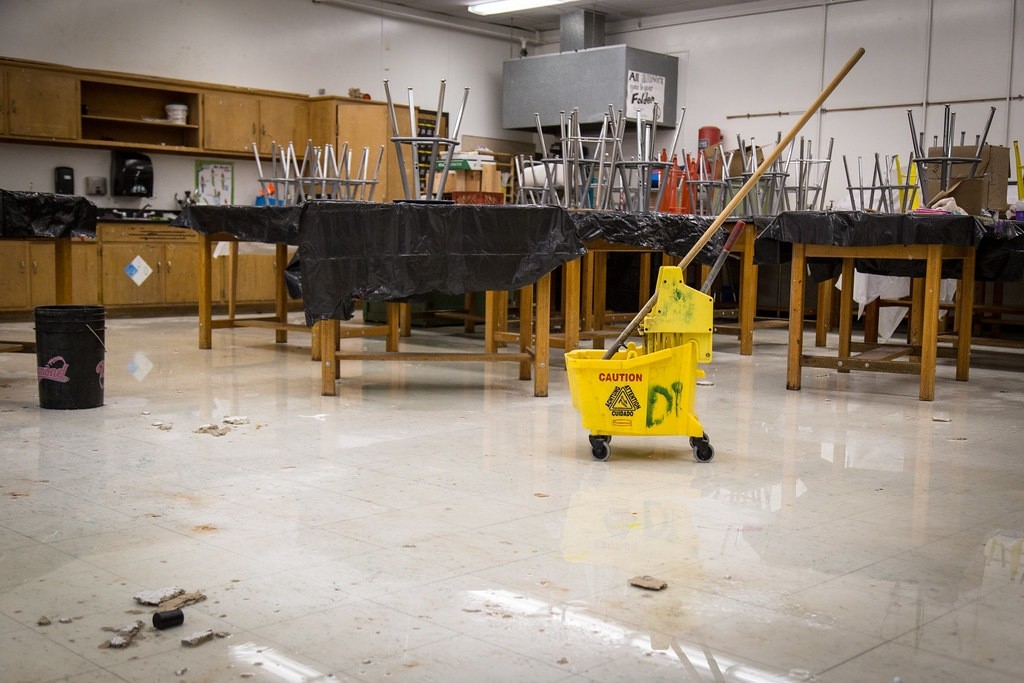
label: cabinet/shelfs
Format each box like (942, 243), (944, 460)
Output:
(1, 56), (516, 317)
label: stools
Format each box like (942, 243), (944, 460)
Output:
(251, 77), (1023, 214)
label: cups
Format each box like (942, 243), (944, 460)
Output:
(165, 104), (189, 124)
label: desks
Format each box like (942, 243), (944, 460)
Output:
(186, 199), (1024, 402)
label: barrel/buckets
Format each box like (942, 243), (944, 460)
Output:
(35, 304), (106, 410)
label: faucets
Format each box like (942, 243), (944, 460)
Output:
(135, 203), (153, 218)
